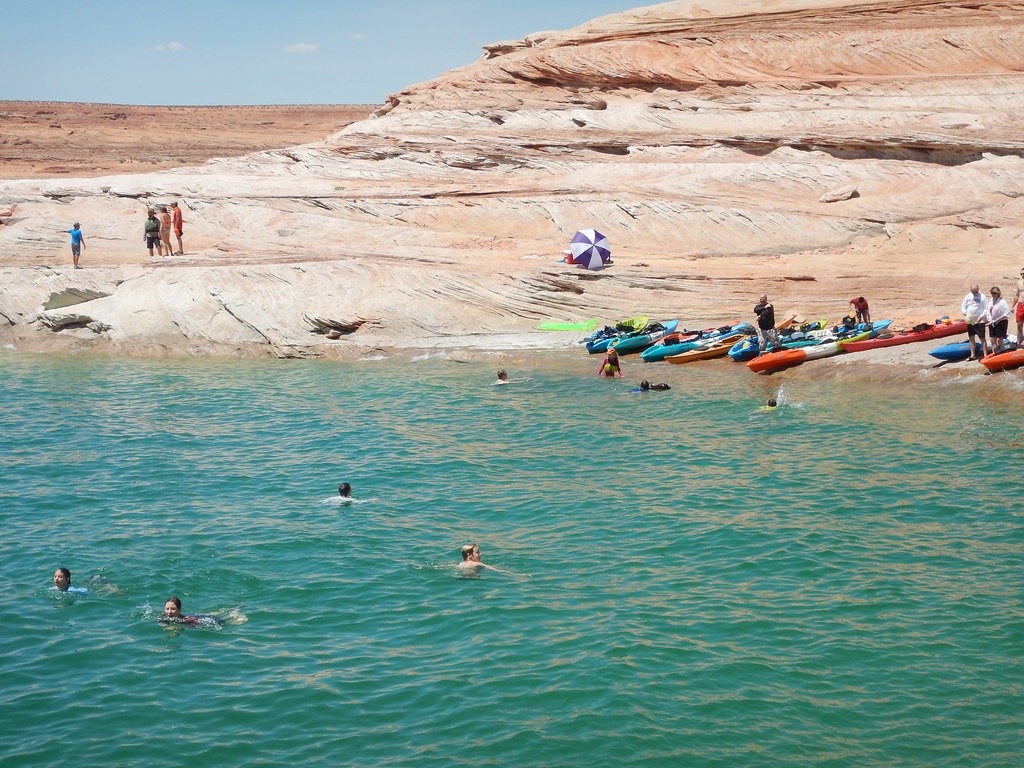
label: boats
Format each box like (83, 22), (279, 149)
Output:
(928, 334), (1024, 362)
(664, 314), (797, 364)
(840, 319), (968, 353)
(746, 331), (872, 373)
(979, 346), (1024, 373)
(654, 327), (717, 344)
(728, 318), (894, 363)
(606, 320), (678, 352)
(585, 314), (649, 354)
(640, 321), (749, 362)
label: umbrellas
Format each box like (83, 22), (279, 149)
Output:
(569, 229), (611, 270)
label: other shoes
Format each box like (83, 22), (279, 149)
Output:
(173, 251), (184, 255)
(74, 263), (83, 269)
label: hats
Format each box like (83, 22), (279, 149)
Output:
(607, 348), (616, 355)
(148, 208), (157, 214)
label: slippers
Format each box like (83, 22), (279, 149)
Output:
(966, 356), (978, 360)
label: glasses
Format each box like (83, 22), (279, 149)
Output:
(990, 291), (999, 295)
(1019, 272), (1024, 277)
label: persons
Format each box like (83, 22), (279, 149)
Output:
(170, 201), (184, 254)
(333, 483), (353, 501)
(985, 286), (1011, 352)
(159, 207), (174, 256)
(496, 369), (509, 383)
(54, 569), (75, 590)
(55, 221), (86, 268)
(143, 208), (162, 256)
(458, 543), (493, 571)
(641, 380), (649, 389)
(850, 297), (870, 324)
(768, 399), (776, 406)
(961, 283), (988, 361)
(164, 596), (181, 617)
(597, 348), (624, 377)
(1011, 268), (1024, 346)
(753, 295), (781, 348)
